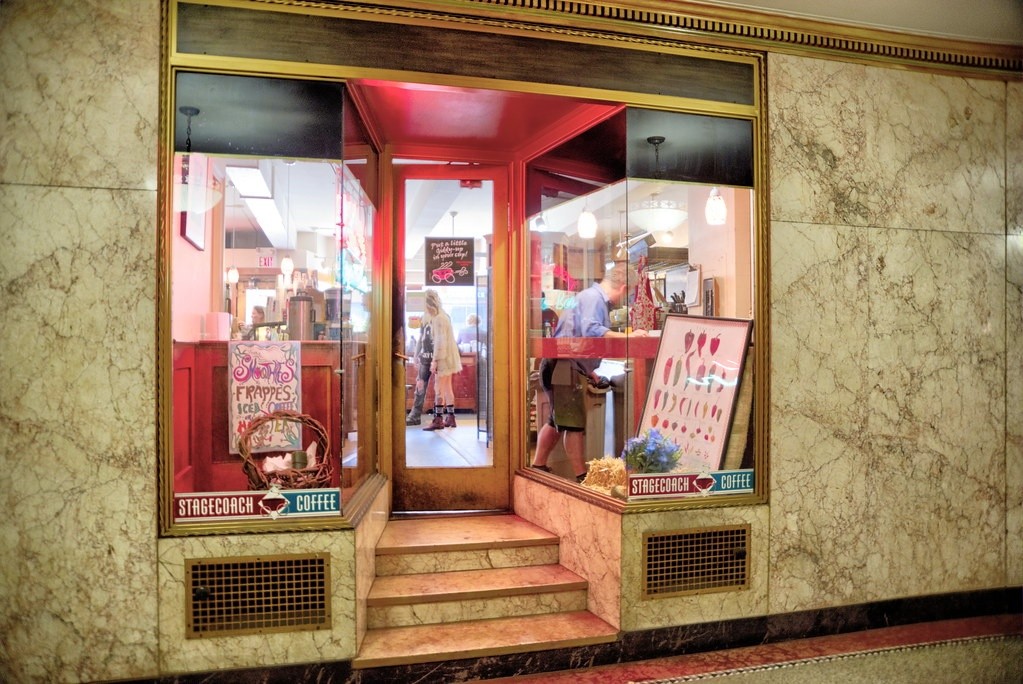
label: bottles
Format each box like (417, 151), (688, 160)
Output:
(410, 335), (416, 352)
(674, 303), (688, 314)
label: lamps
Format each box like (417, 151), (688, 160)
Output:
(578, 196), (597, 238)
(535, 214), (546, 233)
(629, 191), (688, 231)
(281, 159), (295, 277)
(228, 187), (239, 283)
(705, 187), (727, 225)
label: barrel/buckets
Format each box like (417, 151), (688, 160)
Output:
(324, 286), (352, 321)
(289, 295), (315, 340)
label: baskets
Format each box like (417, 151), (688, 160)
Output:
(239, 410), (334, 492)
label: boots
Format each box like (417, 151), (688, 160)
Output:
(444, 414), (456, 427)
(423, 416), (444, 431)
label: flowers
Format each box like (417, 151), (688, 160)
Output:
(620, 428), (683, 472)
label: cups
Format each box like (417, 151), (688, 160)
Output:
(459, 340), (481, 353)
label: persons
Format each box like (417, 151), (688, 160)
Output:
(237, 306), (266, 338)
(531, 263), (652, 483)
(406, 289), (486, 430)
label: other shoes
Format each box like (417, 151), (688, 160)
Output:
(533, 464), (553, 473)
(406, 416), (421, 426)
